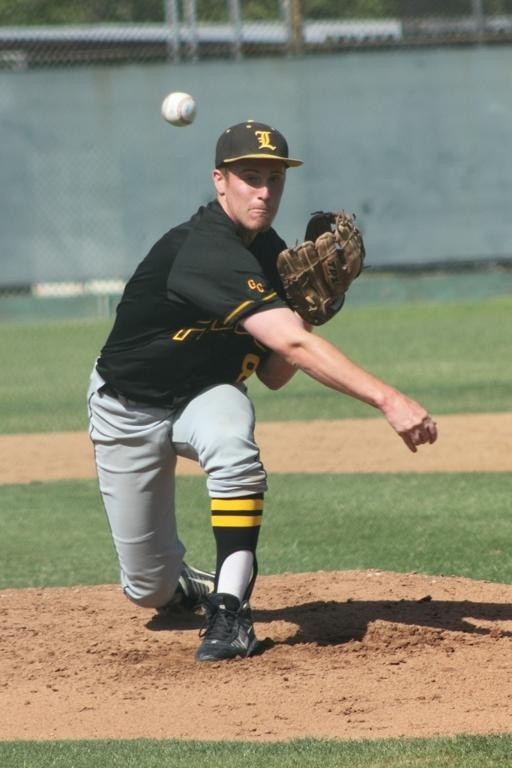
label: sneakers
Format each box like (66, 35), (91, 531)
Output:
(173, 560), (256, 663)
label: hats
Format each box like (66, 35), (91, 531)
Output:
(215, 120), (303, 169)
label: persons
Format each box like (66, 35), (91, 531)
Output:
(87, 118), (438, 657)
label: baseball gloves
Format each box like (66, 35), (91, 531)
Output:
(276, 208), (365, 326)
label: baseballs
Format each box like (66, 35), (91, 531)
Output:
(160, 90), (194, 126)
